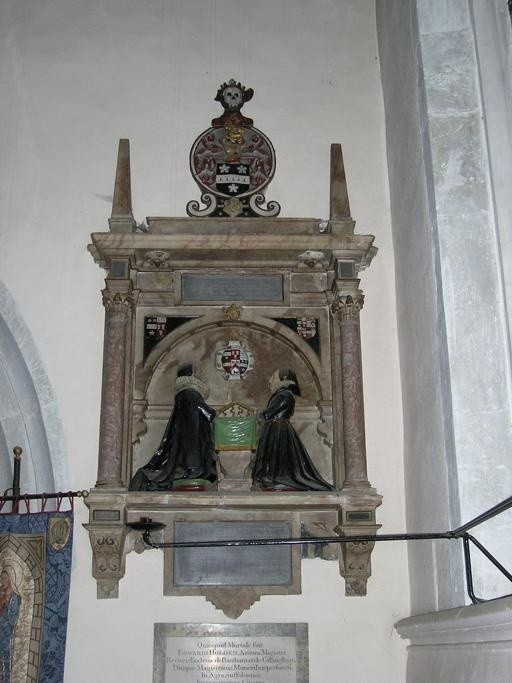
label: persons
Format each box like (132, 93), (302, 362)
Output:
(250, 367), (335, 492)
(129, 362), (227, 491)
